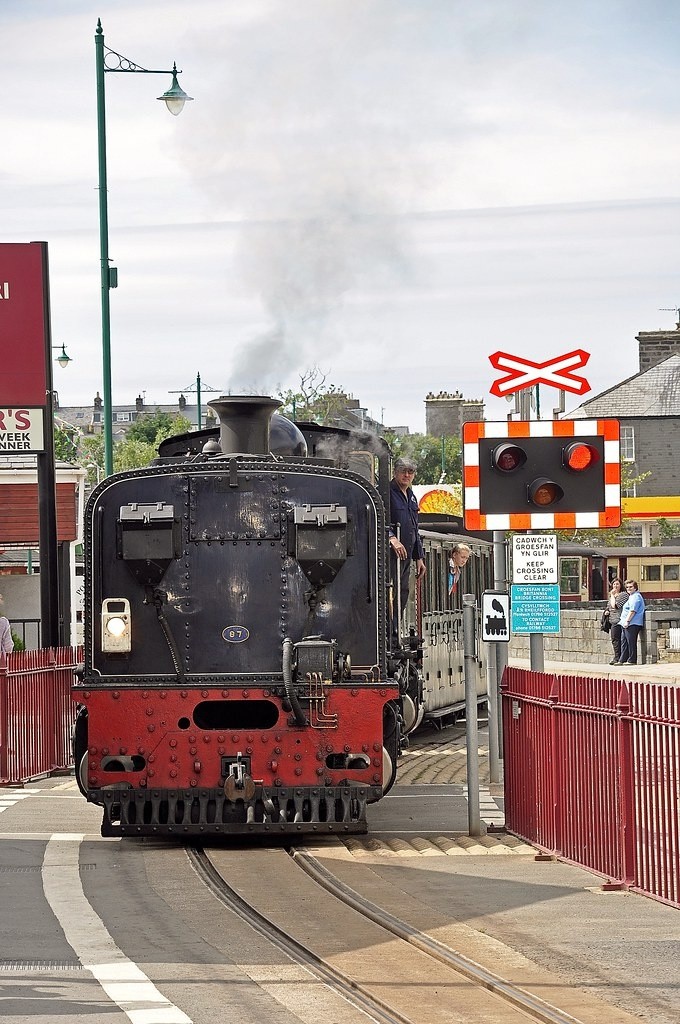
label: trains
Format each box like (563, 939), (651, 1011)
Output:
(73, 394), (680, 838)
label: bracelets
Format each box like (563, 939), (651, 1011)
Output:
(626, 621), (630, 622)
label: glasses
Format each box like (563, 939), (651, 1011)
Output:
(396, 467), (416, 474)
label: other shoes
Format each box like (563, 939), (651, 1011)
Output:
(614, 662), (623, 666)
(623, 661), (637, 665)
(609, 660), (618, 665)
(386, 639), (413, 659)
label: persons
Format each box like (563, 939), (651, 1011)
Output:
(389, 458), (427, 659)
(606, 578), (645, 666)
(566, 564), (603, 600)
(0, 595), (16, 755)
(447, 544), (471, 596)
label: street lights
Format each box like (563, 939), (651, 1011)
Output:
(95, 15), (197, 479)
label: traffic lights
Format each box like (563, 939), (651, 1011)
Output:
(460, 415), (623, 534)
